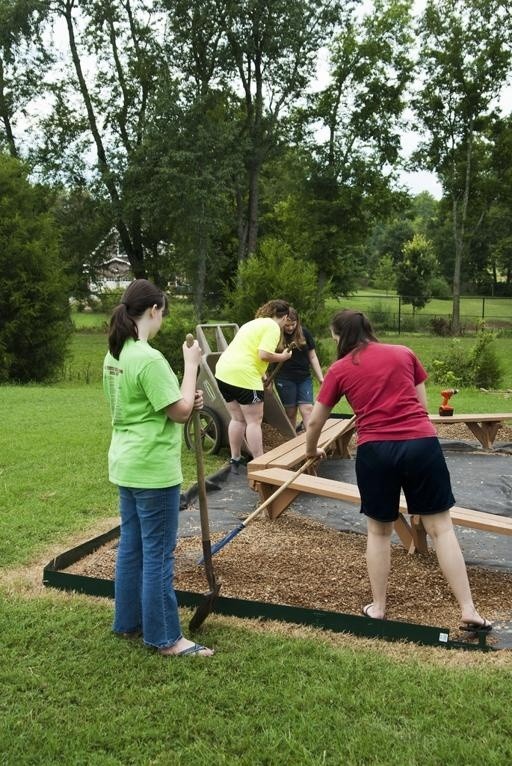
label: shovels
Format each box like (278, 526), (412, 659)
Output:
(185, 333), (222, 632)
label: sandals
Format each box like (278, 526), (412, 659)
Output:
(231, 456), (247, 466)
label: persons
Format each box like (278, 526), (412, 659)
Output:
(305, 307), (495, 632)
(267, 303), (326, 433)
(214, 297), (294, 475)
(104, 279), (217, 658)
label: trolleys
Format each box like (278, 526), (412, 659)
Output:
(185, 323), (297, 456)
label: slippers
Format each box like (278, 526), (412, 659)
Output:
(114, 627), (145, 637)
(362, 604), (387, 621)
(459, 619), (492, 633)
(155, 636), (215, 658)
